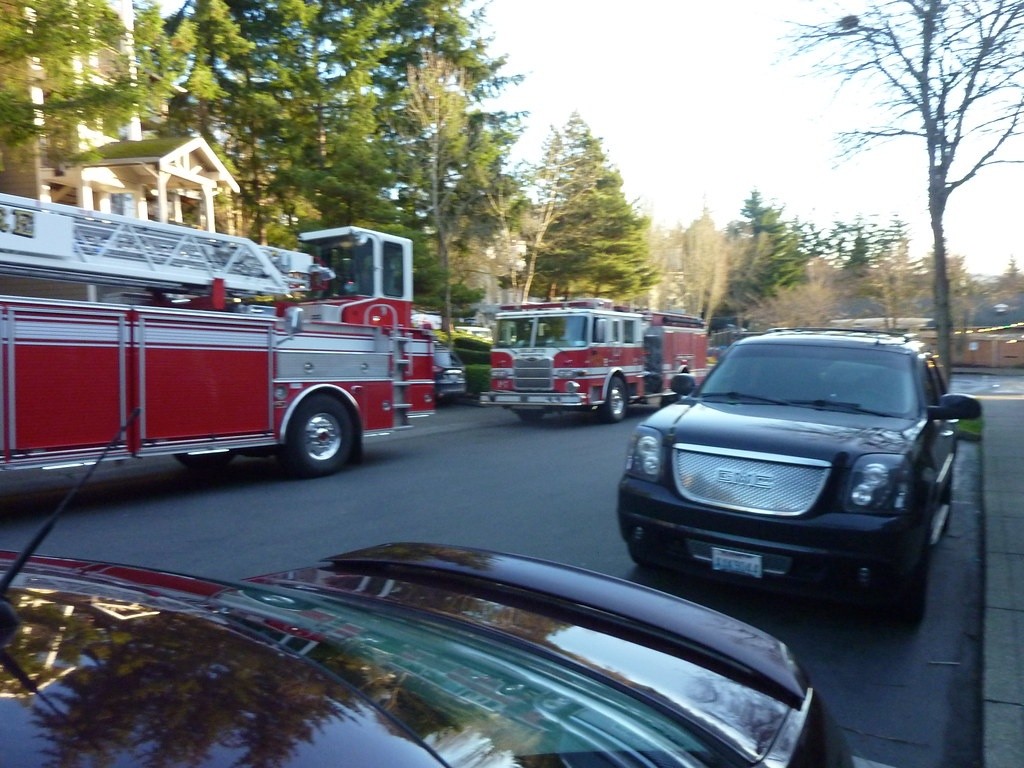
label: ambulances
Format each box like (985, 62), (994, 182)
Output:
(481, 298), (708, 423)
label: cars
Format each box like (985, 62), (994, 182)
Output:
(0, 540), (894, 768)
(613, 327), (983, 624)
(434, 341), (466, 406)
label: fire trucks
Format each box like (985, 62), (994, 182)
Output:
(1, 194), (435, 480)
